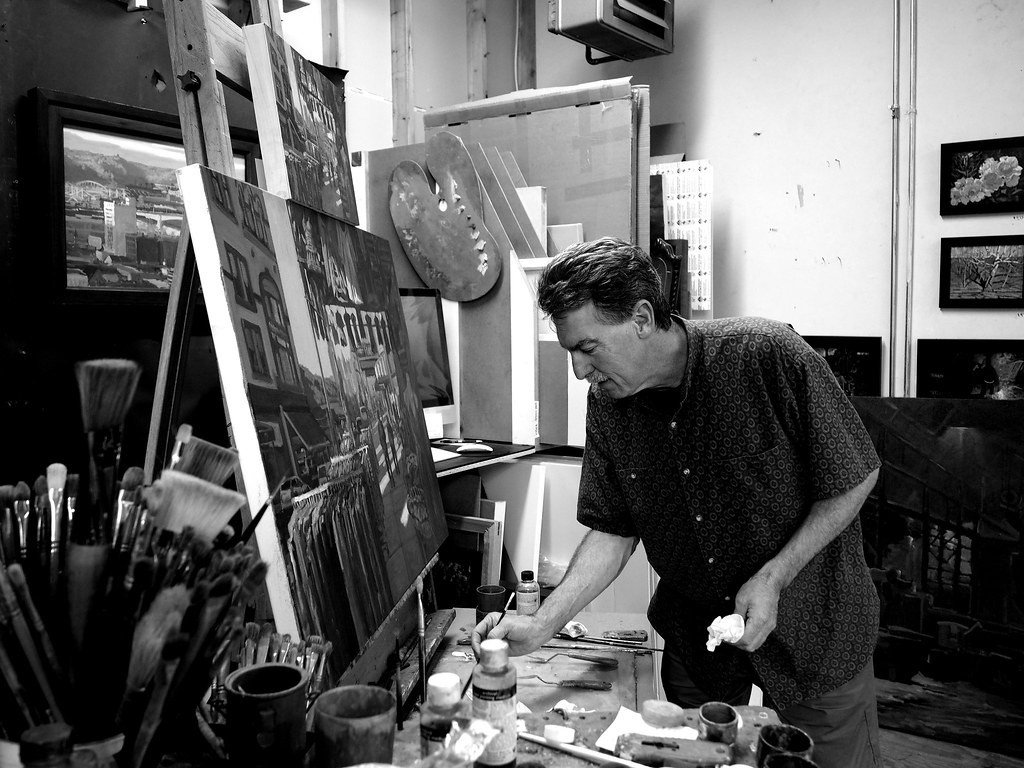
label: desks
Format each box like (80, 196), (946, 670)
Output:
(391, 608), (812, 768)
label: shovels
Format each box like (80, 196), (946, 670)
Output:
(525, 653), (618, 667)
(519, 674), (612, 690)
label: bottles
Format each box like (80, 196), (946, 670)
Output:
(419, 672), (469, 768)
(472, 640), (517, 768)
(516, 571), (540, 615)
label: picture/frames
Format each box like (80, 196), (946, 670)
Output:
(801, 335), (882, 397)
(939, 136), (1024, 216)
(916, 339), (1024, 400)
(938, 234), (1024, 310)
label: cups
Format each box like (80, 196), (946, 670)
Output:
(314, 685), (396, 768)
(698, 702), (739, 745)
(756, 724), (817, 768)
(224, 662), (307, 768)
(476, 585), (506, 625)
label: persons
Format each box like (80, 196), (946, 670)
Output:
(472, 235), (882, 768)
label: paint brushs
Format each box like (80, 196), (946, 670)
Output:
(415, 574), (426, 708)
(0, 357), (338, 768)
(392, 626), (404, 733)
(461, 592), (517, 699)
(541, 633), (664, 656)
